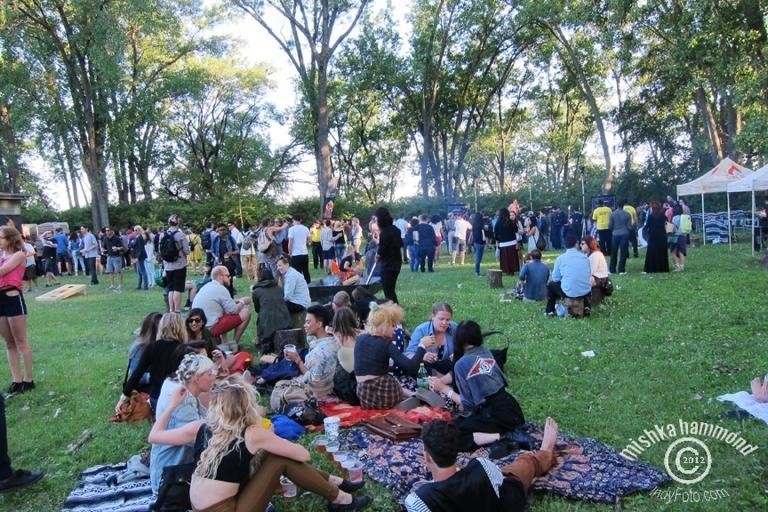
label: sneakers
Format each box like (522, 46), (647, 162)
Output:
(4, 380), (35, 400)
(619, 272), (628, 276)
(2, 382), (21, 397)
(608, 271), (613, 275)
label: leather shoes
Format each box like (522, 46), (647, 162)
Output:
(544, 312), (555, 317)
(339, 479), (366, 492)
(0, 469), (44, 490)
(584, 309), (591, 316)
(328, 493), (372, 512)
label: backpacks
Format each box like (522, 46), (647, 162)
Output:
(160, 229), (180, 263)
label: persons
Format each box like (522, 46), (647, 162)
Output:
(190, 264), (251, 344)
(252, 214), (312, 348)
(143, 354), (374, 512)
(750, 373), (767, 402)
(119, 309), (228, 419)
(20, 224), (164, 290)
(0, 390), (45, 493)
(0, 226), (35, 399)
(286, 286), (525, 430)
(184, 279), (196, 307)
(156, 213), (191, 313)
(184, 223), (257, 280)
(404, 416), (559, 512)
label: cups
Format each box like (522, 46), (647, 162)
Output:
(339, 460), (355, 478)
(324, 442), (339, 459)
(283, 343), (295, 361)
(348, 461), (363, 482)
(280, 478), (297, 503)
(315, 435), (328, 452)
(332, 452), (347, 469)
(324, 416), (340, 437)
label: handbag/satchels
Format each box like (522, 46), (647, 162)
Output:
(482, 331), (510, 372)
(600, 276), (614, 296)
(154, 463), (197, 512)
(116, 386), (150, 421)
(365, 413), (422, 442)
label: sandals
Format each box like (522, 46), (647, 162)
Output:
(679, 264), (684, 271)
(672, 263), (681, 273)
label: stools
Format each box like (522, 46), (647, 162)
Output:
(273, 327), (306, 354)
(564, 297), (584, 317)
(591, 287), (602, 305)
(215, 331), (227, 343)
(288, 311), (306, 328)
(487, 269), (502, 288)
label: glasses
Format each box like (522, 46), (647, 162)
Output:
(188, 317), (201, 323)
(222, 273), (230, 278)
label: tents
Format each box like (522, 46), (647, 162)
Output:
(726, 163), (768, 256)
(676, 157), (756, 245)
